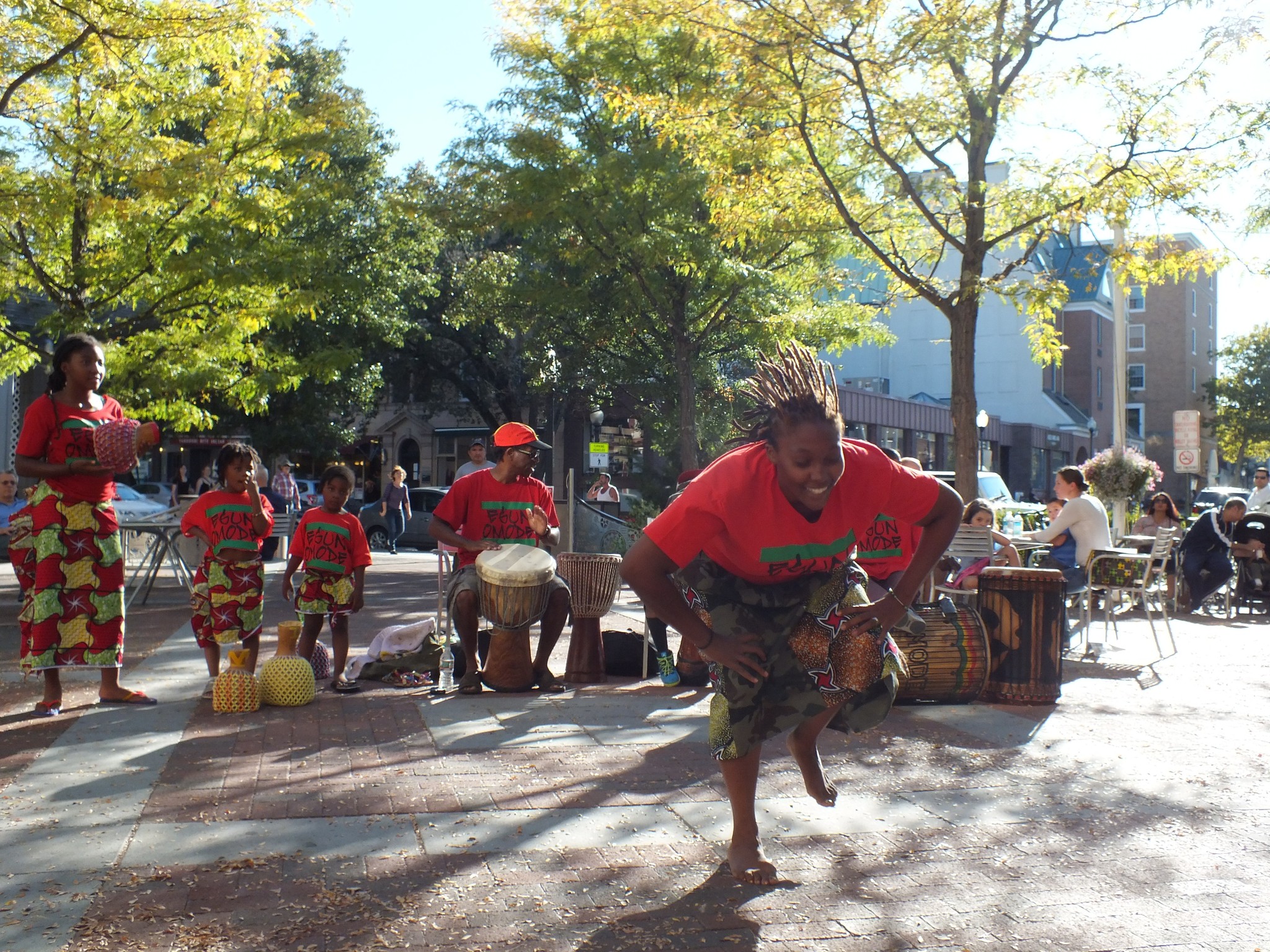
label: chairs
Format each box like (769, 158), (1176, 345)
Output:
(1027, 527), (1232, 659)
(270, 508), (298, 560)
(434, 524), (462, 646)
(928, 524), (1008, 604)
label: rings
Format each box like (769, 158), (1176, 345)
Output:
(873, 616), (879, 627)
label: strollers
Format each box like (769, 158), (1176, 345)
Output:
(1231, 511), (1270, 614)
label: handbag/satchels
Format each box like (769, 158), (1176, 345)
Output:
(602, 628), (659, 676)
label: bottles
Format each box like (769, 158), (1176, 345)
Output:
(1002, 511), (1023, 537)
(438, 647), (454, 692)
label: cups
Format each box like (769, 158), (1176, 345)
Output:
(1256, 549), (1263, 560)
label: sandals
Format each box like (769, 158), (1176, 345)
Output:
(395, 671), (433, 687)
(381, 670), (399, 683)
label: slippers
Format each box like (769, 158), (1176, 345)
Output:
(32, 700), (61, 718)
(331, 677), (360, 690)
(202, 676), (218, 698)
(534, 671), (565, 691)
(459, 671), (483, 693)
(100, 691), (157, 707)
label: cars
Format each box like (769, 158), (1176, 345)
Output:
(358, 486), (452, 551)
(294, 479), (325, 508)
(111, 481), (177, 523)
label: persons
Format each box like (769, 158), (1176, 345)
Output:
(453, 437), (497, 483)
(1131, 492), (1183, 612)
(180, 440), (275, 699)
(618, 341), (1112, 886)
(282, 466), (373, 693)
(1176, 467), (1270, 615)
(379, 465), (412, 554)
(195, 465), (216, 496)
(586, 473), (619, 502)
(172, 464), (194, 507)
(255, 458), (302, 560)
(0, 332), (157, 718)
(427, 422), (571, 695)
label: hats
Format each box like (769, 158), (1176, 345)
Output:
(493, 422), (552, 450)
(468, 438), (484, 448)
(277, 460), (295, 470)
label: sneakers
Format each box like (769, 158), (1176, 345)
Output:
(656, 650), (680, 686)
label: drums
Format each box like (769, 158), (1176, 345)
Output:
(977, 566), (1067, 703)
(475, 543), (557, 693)
(259, 621), (315, 705)
(289, 635), (330, 679)
(874, 606), (990, 701)
(556, 553), (624, 685)
(95, 417), (160, 475)
(213, 649), (258, 712)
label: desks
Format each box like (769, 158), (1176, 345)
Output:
(117, 520), (198, 609)
(1117, 533), (1179, 610)
(1004, 531), (1054, 549)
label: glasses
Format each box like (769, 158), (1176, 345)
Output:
(513, 448), (540, 458)
(0, 481), (16, 485)
(1254, 476), (1267, 480)
(1154, 499), (1168, 504)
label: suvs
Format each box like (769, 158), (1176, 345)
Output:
(1190, 485), (1252, 517)
(923, 469), (1049, 531)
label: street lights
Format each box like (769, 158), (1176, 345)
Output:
(590, 404), (605, 485)
(1085, 415), (1097, 458)
(974, 408), (988, 472)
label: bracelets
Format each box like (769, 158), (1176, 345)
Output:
(69, 463), (75, 475)
(252, 509), (266, 516)
(696, 629), (714, 650)
(534, 525), (551, 539)
(888, 587), (909, 611)
(1234, 541), (1237, 549)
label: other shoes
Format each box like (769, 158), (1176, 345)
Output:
(1091, 594), (1099, 608)
(1165, 599), (1175, 610)
(1133, 598), (1145, 610)
(1063, 630), (1070, 648)
(893, 608), (926, 633)
(1071, 594), (1079, 607)
(391, 551), (397, 554)
